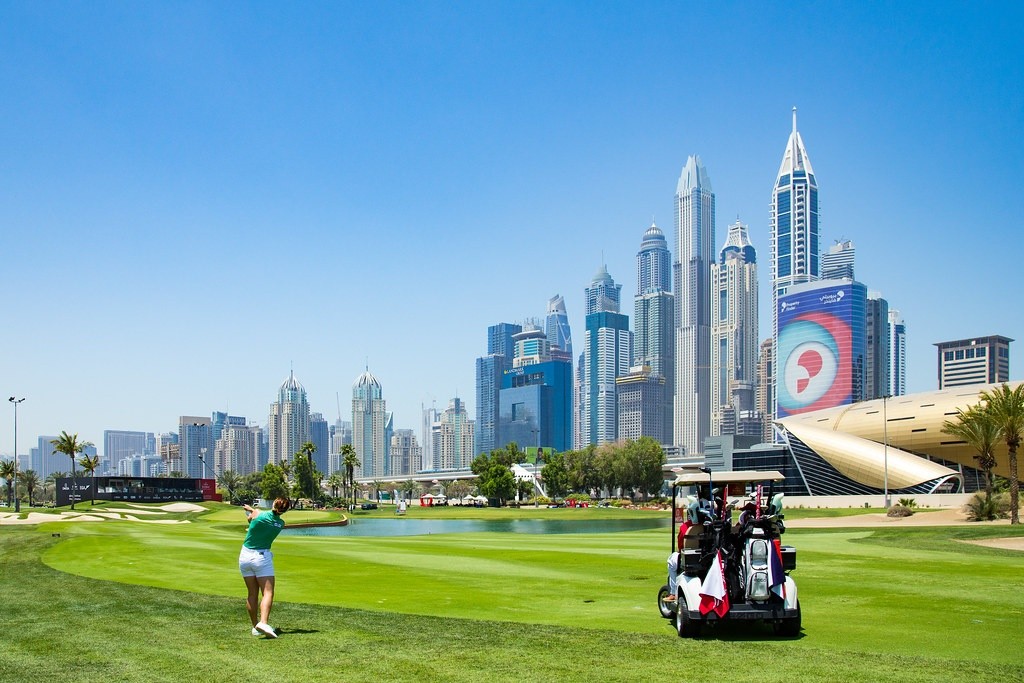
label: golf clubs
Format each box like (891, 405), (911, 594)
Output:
(197, 454), (248, 506)
(699, 466), (724, 521)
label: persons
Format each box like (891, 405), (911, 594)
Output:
(662, 498), (711, 603)
(238, 497), (289, 638)
(445, 501), (448, 506)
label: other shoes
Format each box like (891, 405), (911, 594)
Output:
(663, 595), (676, 602)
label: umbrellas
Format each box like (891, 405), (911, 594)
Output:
(421, 493), (447, 499)
(464, 495), (488, 501)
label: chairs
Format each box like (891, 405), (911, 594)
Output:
(683, 524), (704, 550)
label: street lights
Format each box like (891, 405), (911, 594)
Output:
(8, 397), (26, 508)
(883, 395), (894, 508)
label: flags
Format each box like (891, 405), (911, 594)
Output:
(768, 539), (786, 598)
(700, 550), (730, 617)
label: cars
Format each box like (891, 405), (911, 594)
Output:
(0, 499), (57, 509)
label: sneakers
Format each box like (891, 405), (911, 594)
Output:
(251, 626), (275, 636)
(255, 622), (277, 638)
